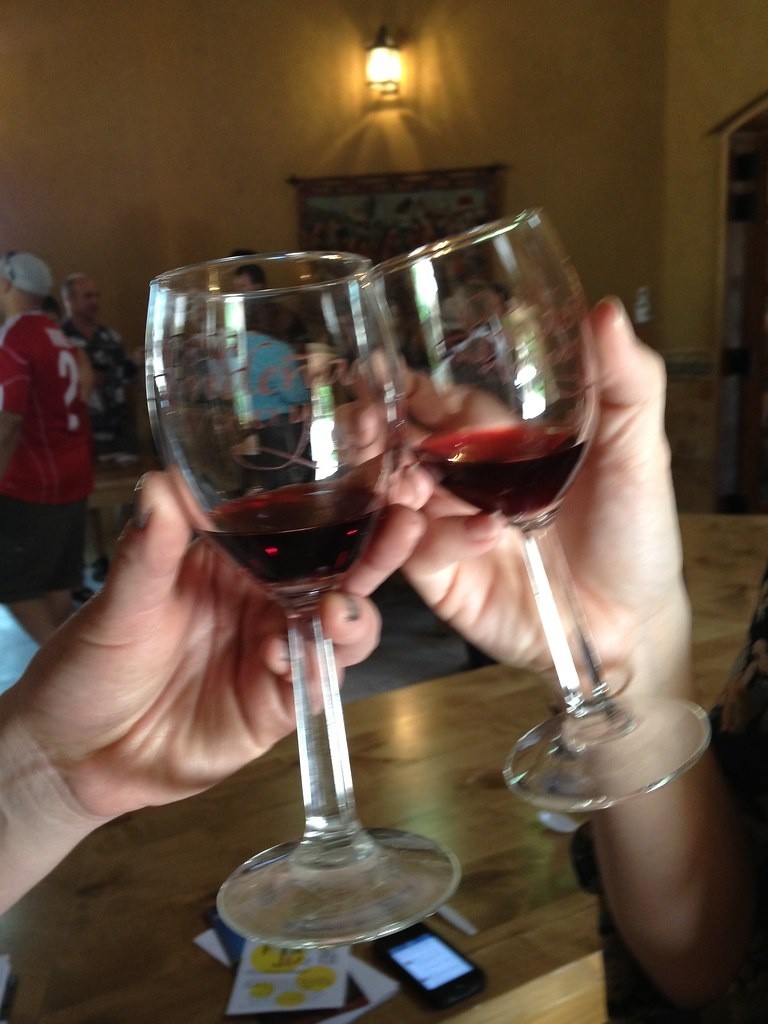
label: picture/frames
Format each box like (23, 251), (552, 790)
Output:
(286, 163), (506, 289)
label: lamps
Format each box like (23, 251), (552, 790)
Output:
(366, 21), (407, 88)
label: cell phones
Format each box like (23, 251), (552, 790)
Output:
(370, 918), (489, 1011)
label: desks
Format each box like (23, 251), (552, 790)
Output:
(0, 507), (768, 1023)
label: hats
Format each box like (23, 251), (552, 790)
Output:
(0, 251), (52, 296)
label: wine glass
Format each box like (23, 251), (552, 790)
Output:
(360, 208), (712, 814)
(144, 250), (464, 950)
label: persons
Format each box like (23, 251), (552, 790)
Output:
(0, 252), (768, 1024)
